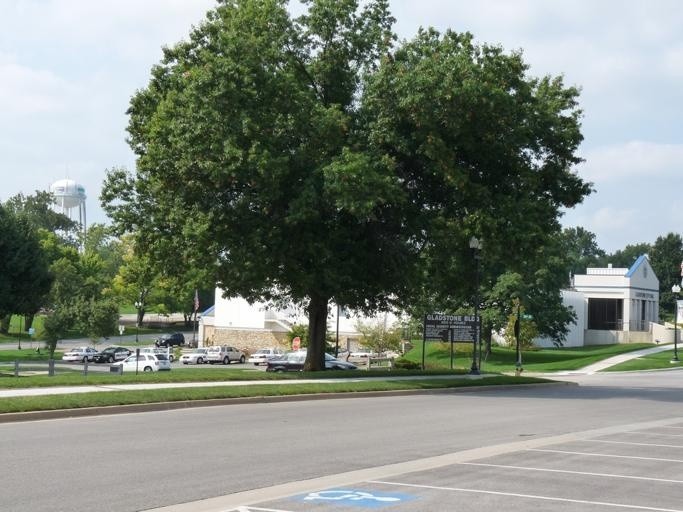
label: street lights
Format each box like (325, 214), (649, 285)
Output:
(670, 282), (682, 365)
(134, 301), (142, 375)
(466, 232), (485, 377)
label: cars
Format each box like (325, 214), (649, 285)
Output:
(154, 331), (185, 348)
(62, 346), (102, 362)
(112, 352), (171, 373)
(93, 346), (132, 363)
(248, 348), (285, 366)
(180, 347), (210, 364)
(266, 350), (357, 374)
(347, 353), (370, 365)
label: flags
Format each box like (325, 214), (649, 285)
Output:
(194, 290), (199, 311)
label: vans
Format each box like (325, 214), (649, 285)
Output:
(206, 345), (246, 365)
